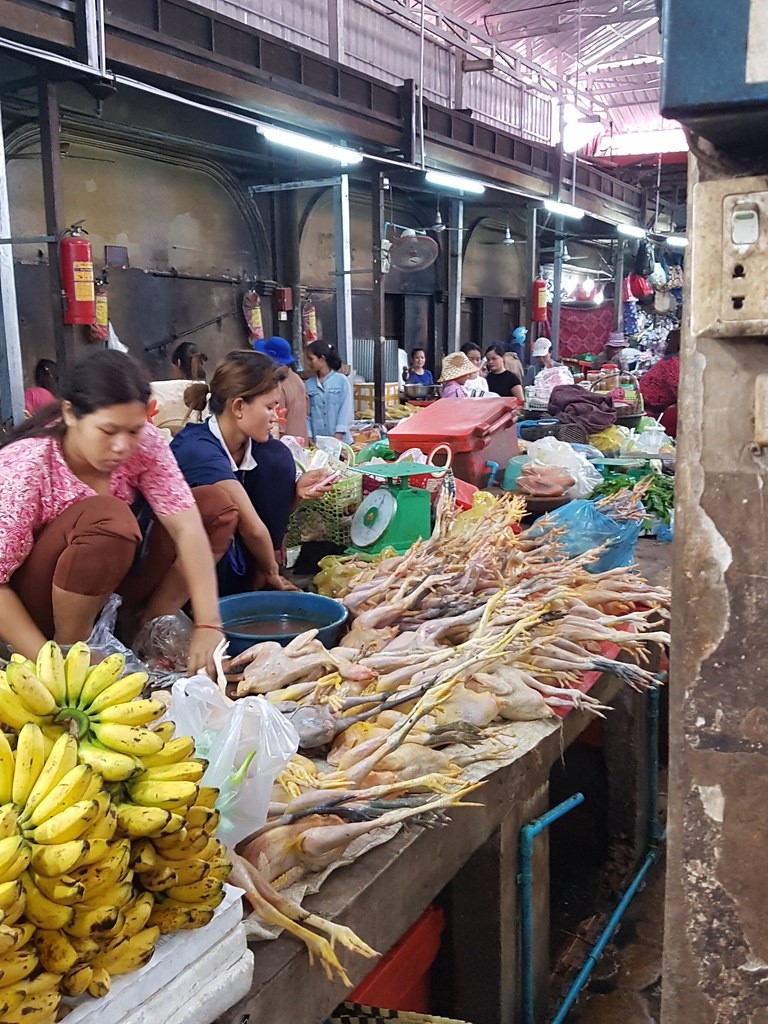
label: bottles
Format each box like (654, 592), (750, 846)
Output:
(572, 364), (620, 391)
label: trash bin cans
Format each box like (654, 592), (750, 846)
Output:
(246, 295), (266, 345)
(385, 394), (521, 489)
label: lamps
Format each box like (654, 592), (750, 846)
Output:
(561, 245), (570, 261)
(666, 237), (688, 246)
(502, 222), (514, 245)
(425, 170), (485, 196)
(255, 125), (364, 166)
(542, 200), (585, 219)
(617, 225), (645, 238)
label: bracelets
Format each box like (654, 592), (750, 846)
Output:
(192, 624), (224, 634)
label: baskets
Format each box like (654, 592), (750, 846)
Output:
(589, 370), (644, 416)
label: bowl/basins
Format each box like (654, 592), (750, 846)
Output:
(500, 454), (528, 492)
(218, 591), (349, 661)
(402, 384), (442, 398)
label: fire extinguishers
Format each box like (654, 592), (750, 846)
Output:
(302, 302), (316, 346)
(60, 220), (96, 327)
(89, 276), (110, 343)
(533, 276), (550, 325)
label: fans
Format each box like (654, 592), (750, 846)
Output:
(381, 229), (438, 273)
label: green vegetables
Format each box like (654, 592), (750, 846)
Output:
(590, 470), (674, 534)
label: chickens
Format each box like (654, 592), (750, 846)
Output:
(208, 469), (671, 991)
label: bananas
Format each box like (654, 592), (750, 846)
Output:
(0, 641), (233, 1024)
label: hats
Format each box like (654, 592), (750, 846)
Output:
(437, 352), (480, 383)
(604, 332), (630, 347)
(254, 337), (296, 365)
(532, 337), (552, 356)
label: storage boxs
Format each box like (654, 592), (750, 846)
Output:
(386, 396), (521, 489)
(343, 900), (443, 1013)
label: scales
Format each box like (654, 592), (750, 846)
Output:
(349, 459), (447, 563)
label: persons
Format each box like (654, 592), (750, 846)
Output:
(401, 337), (564, 401)
(0, 349), (228, 682)
(129, 350), (341, 598)
(253, 336), (311, 446)
(171, 342), (208, 380)
(24, 359), (61, 420)
(596, 333), (631, 376)
(304, 340), (355, 462)
(639, 330), (681, 440)
